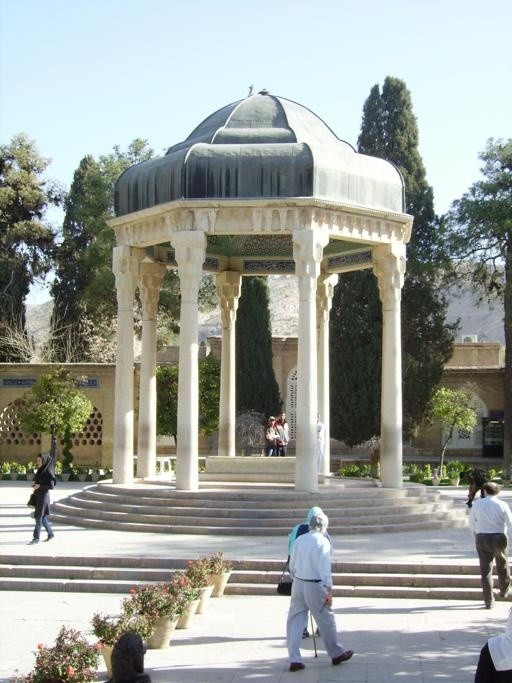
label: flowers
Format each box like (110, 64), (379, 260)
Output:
(5, 549), (235, 682)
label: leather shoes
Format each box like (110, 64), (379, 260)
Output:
(332, 650), (354, 665)
(289, 663), (305, 672)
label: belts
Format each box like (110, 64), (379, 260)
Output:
(295, 579), (322, 583)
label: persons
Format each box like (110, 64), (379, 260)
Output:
(287, 504), (334, 638)
(467, 480), (511, 610)
(27, 453), (56, 546)
(105, 630), (151, 682)
(458, 466), (489, 507)
(264, 415), (281, 456)
(285, 512), (355, 672)
(473, 601), (511, 682)
(275, 411), (291, 456)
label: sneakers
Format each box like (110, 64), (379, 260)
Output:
(301, 627), (321, 637)
(500, 584), (510, 599)
(485, 596), (494, 609)
(27, 538), (39, 544)
(44, 535), (54, 542)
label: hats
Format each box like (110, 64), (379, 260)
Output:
(269, 416), (275, 421)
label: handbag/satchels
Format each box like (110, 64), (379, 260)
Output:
(27, 493), (35, 508)
(277, 581), (292, 596)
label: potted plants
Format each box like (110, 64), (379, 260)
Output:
(406, 458), (465, 487)
